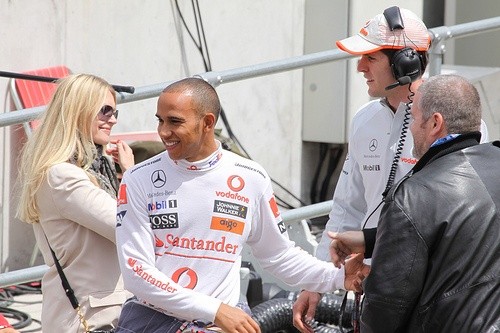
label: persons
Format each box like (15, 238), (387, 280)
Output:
(326, 75), (500, 333)
(118, 78), (371, 333)
(291, 6), (488, 333)
(16, 74), (136, 333)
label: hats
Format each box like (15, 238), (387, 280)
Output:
(337, 7), (432, 56)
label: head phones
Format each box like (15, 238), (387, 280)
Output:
(383, 6), (421, 82)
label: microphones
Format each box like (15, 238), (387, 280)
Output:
(384, 76), (411, 91)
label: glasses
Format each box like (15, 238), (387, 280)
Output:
(97, 104), (118, 118)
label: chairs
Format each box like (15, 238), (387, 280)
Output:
(8, 65), (72, 267)
